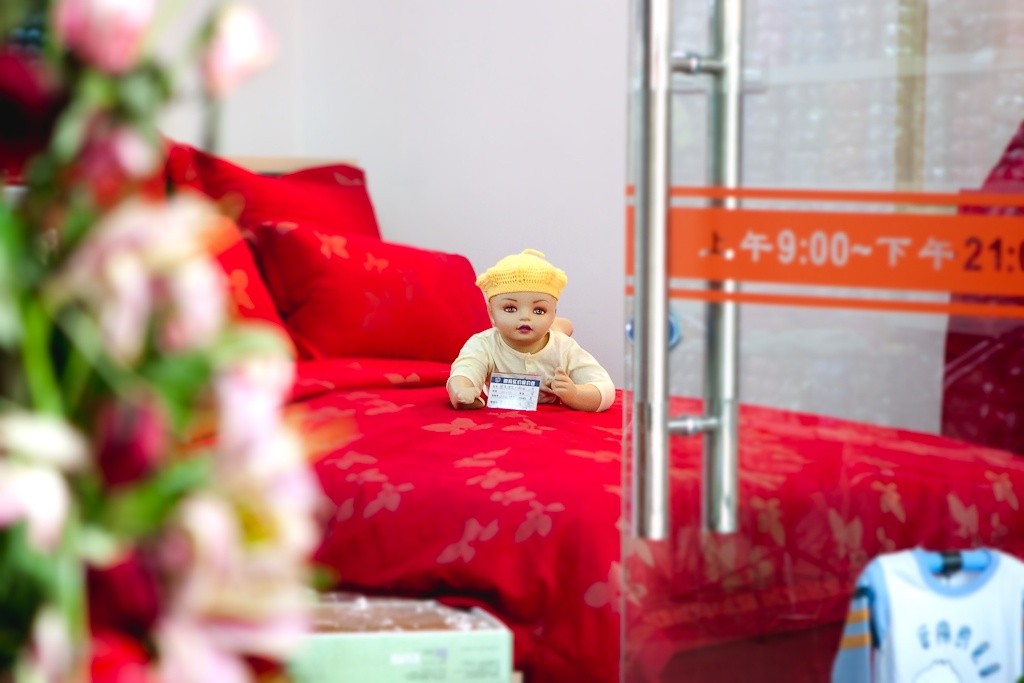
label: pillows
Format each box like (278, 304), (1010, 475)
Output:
(1, 140), (492, 366)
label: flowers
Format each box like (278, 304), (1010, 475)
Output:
(0, 0), (333, 683)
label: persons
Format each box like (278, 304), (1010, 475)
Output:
(448, 250), (617, 412)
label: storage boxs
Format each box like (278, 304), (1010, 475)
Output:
(280, 591), (513, 682)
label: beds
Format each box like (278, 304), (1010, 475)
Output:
(0, 137), (1024, 683)
